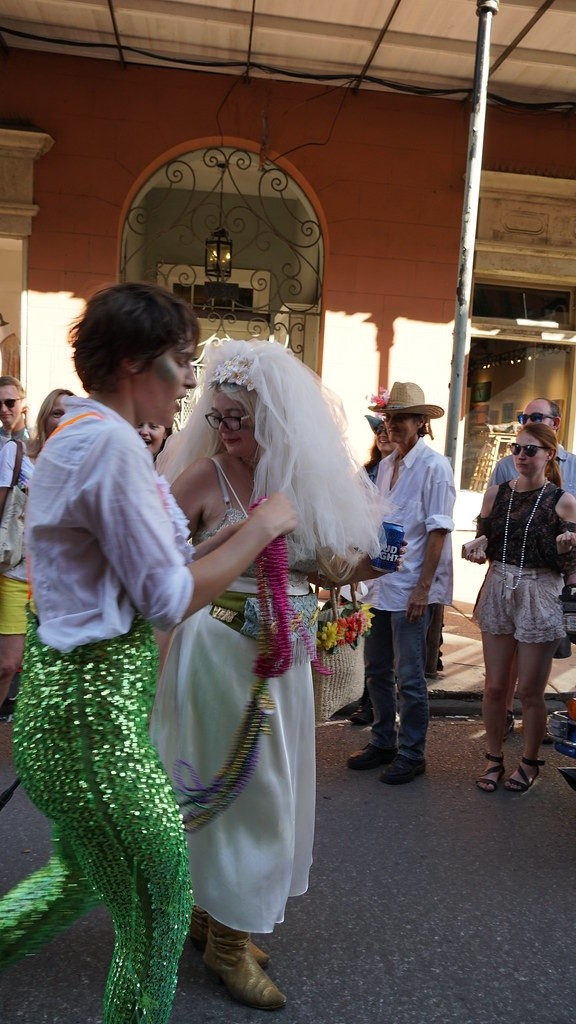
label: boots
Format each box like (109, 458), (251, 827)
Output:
(190, 905), (270, 967)
(350, 681), (374, 724)
(202, 914), (287, 1009)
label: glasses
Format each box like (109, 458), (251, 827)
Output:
(517, 413), (554, 424)
(205, 411), (250, 431)
(375, 426), (387, 436)
(510, 443), (548, 457)
(0, 399), (20, 409)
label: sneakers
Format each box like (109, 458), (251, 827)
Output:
(347, 743), (398, 770)
(381, 754), (425, 785)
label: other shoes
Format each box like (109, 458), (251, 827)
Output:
(503, 710), (514, 741)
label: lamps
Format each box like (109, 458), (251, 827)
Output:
(202, 161), (235, 285)
(465, 343), (571, 371)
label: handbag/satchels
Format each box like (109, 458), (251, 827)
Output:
(559, 584), (576, 645)
(0, 439), (28, 575)
(311, 583), (366, 723)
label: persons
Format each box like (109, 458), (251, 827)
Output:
(0, 374), (174, 708)
(347, 382), (456, 784)
(488, 398), (576, 742)
(465, 424), (576, 792)
(157, 337), (407, 1010)
(0, 281), (298, 1024)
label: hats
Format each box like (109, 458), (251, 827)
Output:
(364, 414), (384, 433)
(368, 382), (444, 419)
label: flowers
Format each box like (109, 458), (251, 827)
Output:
(309, 599), (376, 655)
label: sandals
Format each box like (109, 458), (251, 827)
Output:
(476, 752), (505, 792)
(503, 757), (545, 792)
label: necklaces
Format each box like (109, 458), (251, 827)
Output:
(171, 497), (336, 831)
(502, 476), (548, 590)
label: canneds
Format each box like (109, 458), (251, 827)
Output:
(371, 520), (405, 572)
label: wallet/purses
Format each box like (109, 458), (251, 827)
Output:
(462, 535), (488, 558)
